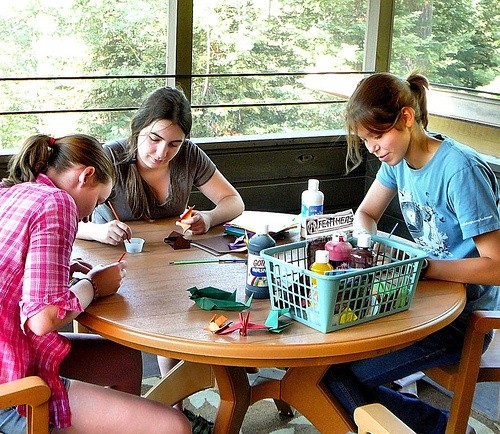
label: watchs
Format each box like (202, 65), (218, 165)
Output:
(418, 258), (429, 281)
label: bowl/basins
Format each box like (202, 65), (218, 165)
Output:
(124, 238), (144, 253)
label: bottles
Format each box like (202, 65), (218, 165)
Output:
(309, 250), (333, 307)
(245, 224), (276, 299)
(325, 231), (374, 320)
(301, 179), (324, 238)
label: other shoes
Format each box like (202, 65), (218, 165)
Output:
(183, 408), (214, 434)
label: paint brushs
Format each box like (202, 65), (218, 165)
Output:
(387, 222), (398, 238)
(219, 260), (246, 262)
(179, 205), (196, 222)
(118, 252), (126, 262)
(169, 260), (219, 264)
(108, 200), (131, 243)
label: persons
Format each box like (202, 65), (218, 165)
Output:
(75, 86), (259, 434)
(321, 73), (500, 434)
(0, 134), (195, 433)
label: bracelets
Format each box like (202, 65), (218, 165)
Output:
(81, 278), (98, 302)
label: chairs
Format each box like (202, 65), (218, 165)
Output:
(421, 309), (500, 434)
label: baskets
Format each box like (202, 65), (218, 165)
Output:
(260, 230), (429, 333)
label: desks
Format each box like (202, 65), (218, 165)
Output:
(70, 211), (467, 434)
